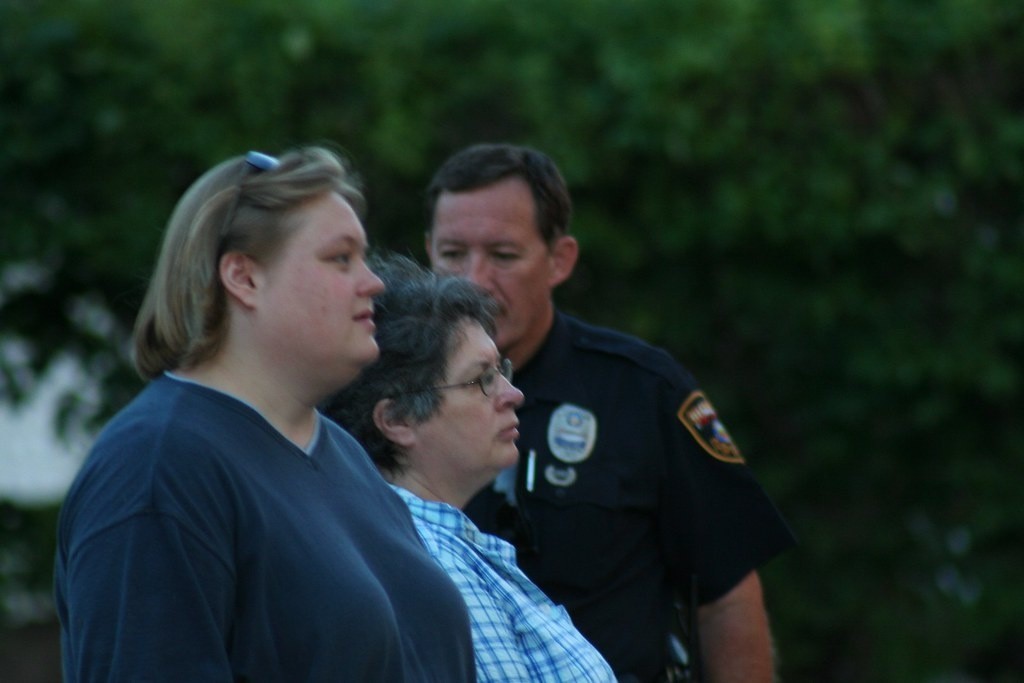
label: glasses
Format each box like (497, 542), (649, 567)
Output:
(218, 150), (280, 248)
(402, 357), (512, 396)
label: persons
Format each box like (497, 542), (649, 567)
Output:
(52, 147), (476, 683)
(316, 254), (617, 683)
(423, 146), (776, 683)
